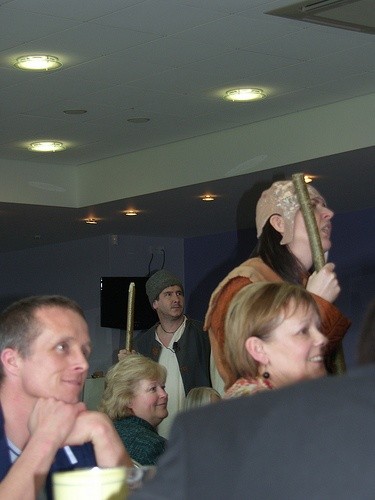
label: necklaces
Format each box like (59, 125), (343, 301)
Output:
(160, 316), (185, 333)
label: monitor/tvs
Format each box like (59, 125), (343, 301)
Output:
(100, 276), (160, 331)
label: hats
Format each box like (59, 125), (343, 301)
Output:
(255, 181), (326, 245)
(146, 270), (184, 306)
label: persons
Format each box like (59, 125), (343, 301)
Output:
(202, 180), (354, 400)
(0, 270), (375, 500)
